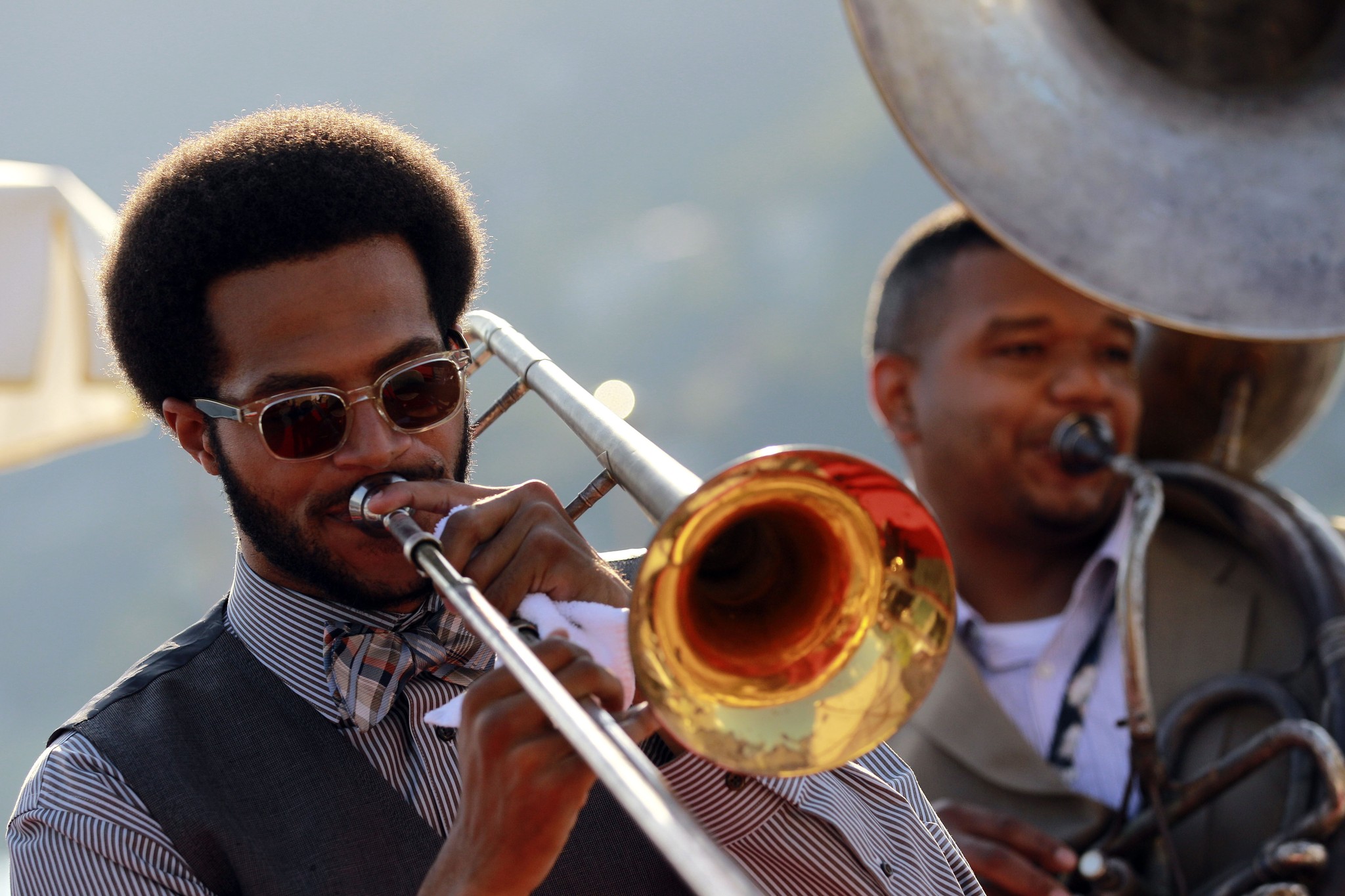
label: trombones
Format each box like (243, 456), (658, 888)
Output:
(342, 307), (964, 895)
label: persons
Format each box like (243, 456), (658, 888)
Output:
(0, 105), (991, 896)
(839, 201), (1345, 896)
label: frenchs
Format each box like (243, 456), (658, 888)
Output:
(834, 0), (1345, 896)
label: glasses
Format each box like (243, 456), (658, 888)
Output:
(186, 329), (474, 464)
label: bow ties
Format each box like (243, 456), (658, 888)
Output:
(322, 603), (496, 735)
